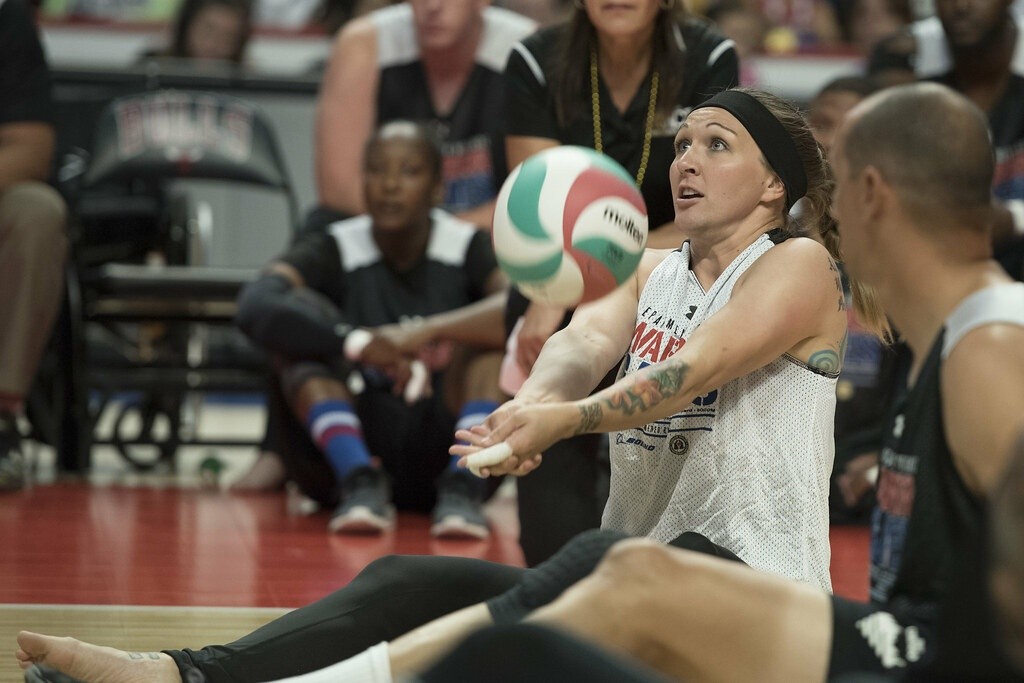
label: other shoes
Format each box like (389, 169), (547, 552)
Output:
(0, 416), (25, 492)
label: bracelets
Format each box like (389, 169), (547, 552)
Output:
(342, 327), (372, 361)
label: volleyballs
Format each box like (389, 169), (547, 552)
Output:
(489, 144), (651, 308)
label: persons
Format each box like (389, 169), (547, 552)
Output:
(502, 0), (740, 569)
(680, 0), (1024, 523)
(16, 89), (894, 683)
(1, 1), (541, 494)
(233, 119), (508, 537)
(411, 81), (1024, 683)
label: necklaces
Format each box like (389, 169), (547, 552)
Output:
(591, 47), (658, 187)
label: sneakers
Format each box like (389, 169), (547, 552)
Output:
(430, 482), (488, 541)
(327, 467), (391, 533)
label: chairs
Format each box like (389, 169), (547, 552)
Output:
(68, 96), (305, 475)
